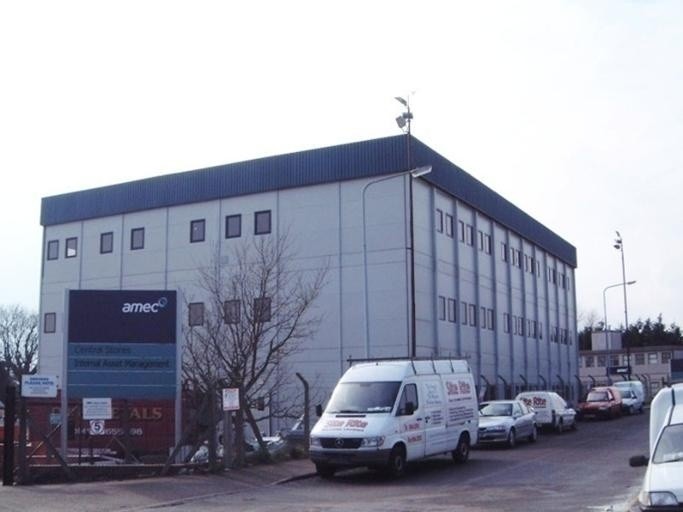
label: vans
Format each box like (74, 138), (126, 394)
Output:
(514, 380), (648, 435)
(307, 356), (481, 480)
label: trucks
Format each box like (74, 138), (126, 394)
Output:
(630, 382), (683, 512)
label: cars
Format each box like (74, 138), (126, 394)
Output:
(480, 396), (538, 448)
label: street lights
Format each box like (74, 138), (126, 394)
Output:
(602, 279), (639, 362)
(359, 163), (434, 356)
